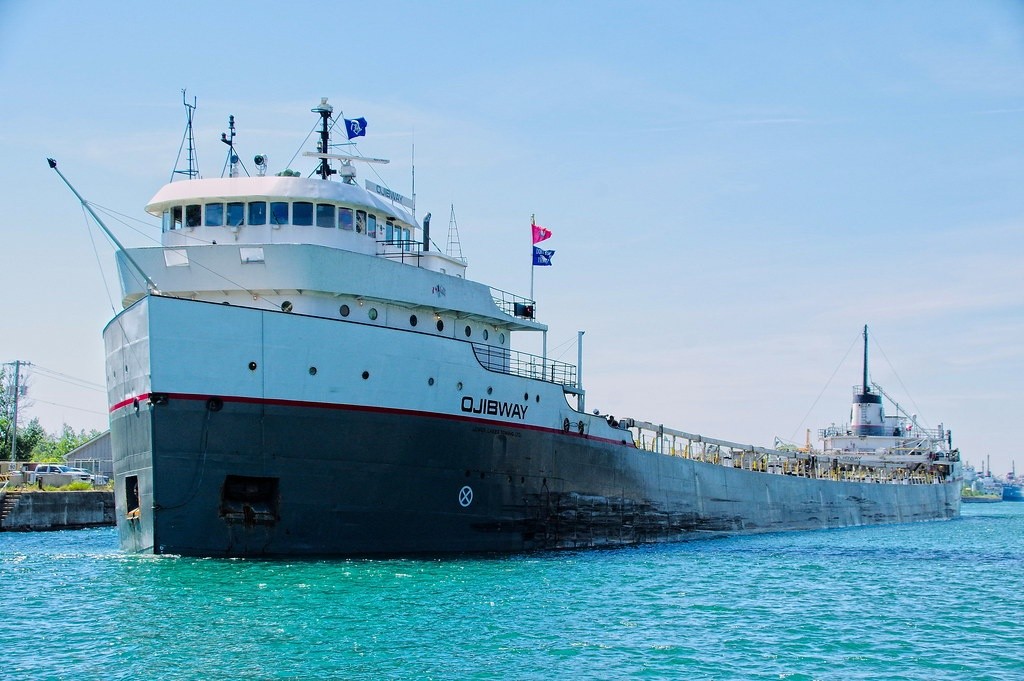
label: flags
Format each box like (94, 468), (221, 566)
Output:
(345, 116), (367, 140)
(532, 224), (553, 243)
(533, 245), (556, 266)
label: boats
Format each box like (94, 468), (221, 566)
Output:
(961, 454), (1024, 504)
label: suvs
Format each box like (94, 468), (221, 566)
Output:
(33, 464), (108, 487)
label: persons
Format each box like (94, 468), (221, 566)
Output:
(607, 415), (620, 428)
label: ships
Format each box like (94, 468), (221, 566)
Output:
(45, 89), (961, 558)
